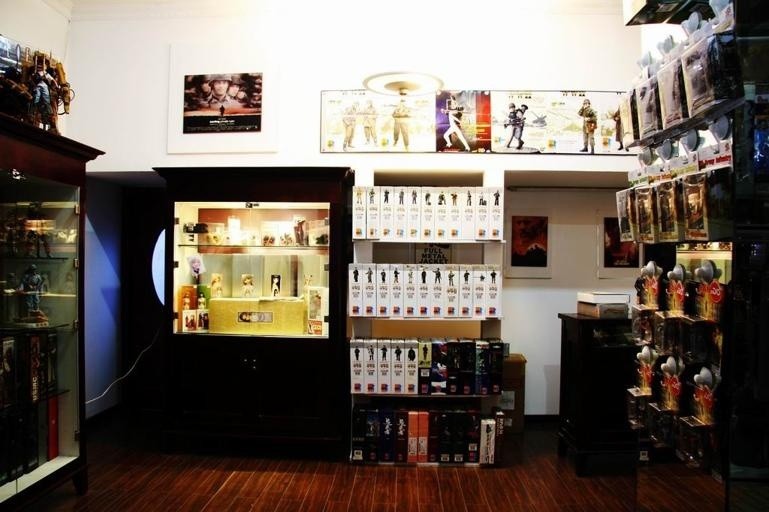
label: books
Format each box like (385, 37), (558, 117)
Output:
(350, 185), (503, 468)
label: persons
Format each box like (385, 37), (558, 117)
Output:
(184, 75), (262, 118)
(320, 89), (630, 154)
(0, 172), (76, 483)
(8, 47), (73, 139)
(515, 216), (544, 239)
(175, 200), (329, 335)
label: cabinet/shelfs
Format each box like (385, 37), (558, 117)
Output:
(0, 112), (106, 512)
(151, 167), (354, 462)
(349, 238), (507, 469)
(500, 353), (527, 436)
(559, 312), (634, 472)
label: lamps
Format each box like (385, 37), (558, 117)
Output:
(363, 72), (444, 117)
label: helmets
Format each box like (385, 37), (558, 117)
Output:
(196, 75), (242, 86)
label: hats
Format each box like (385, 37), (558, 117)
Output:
(583, 99), (589, 104)
(508, 103), (528, 114)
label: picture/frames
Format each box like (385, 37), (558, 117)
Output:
(595, 208), (642, 280)
(166, 41), (283, 155)
(504, 206), (554, 278)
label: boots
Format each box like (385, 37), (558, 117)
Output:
(579, 145), (587, 152)
(618, 142), (622, 150)
(590, 147), (594, 154)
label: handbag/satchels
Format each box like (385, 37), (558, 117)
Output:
(586, 123), (597, 132)
